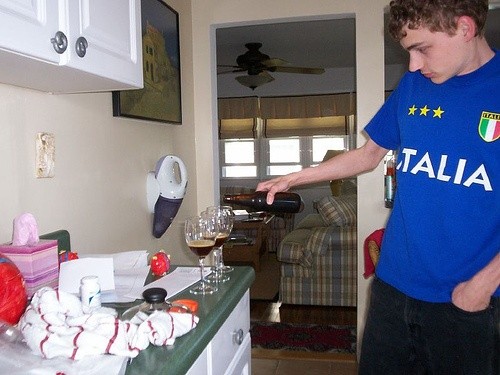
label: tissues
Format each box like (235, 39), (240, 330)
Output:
(0, 212), (59, 300)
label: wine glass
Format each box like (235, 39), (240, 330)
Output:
(185, 215), (219, 295)
(199, 206), (235, 283)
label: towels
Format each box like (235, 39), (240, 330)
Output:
(17, 286), (200, 360)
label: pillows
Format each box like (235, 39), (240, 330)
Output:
(314, 180), (356, 227)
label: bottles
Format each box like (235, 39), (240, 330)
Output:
(223, 190), (302, 213)
(80, 275), (101, 310)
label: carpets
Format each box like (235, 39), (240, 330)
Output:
(250, 320), (357, 355)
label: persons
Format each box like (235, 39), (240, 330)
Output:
(255, 0), (500, 375)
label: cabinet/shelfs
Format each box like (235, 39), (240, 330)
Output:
(0, 0), (144, 95)
(187, 288), (253, 375)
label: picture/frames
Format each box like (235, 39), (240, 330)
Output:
(113, 0), (182, 125)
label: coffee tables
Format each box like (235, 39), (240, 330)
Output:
(224, 212), (275, 265)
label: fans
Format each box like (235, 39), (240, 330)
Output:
(217, 42), (327, 77)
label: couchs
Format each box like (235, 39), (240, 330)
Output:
(220, 186), (294, 252)
(276, 200), (357, 307)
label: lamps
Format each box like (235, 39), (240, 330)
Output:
(235, 63), (276, 92)
